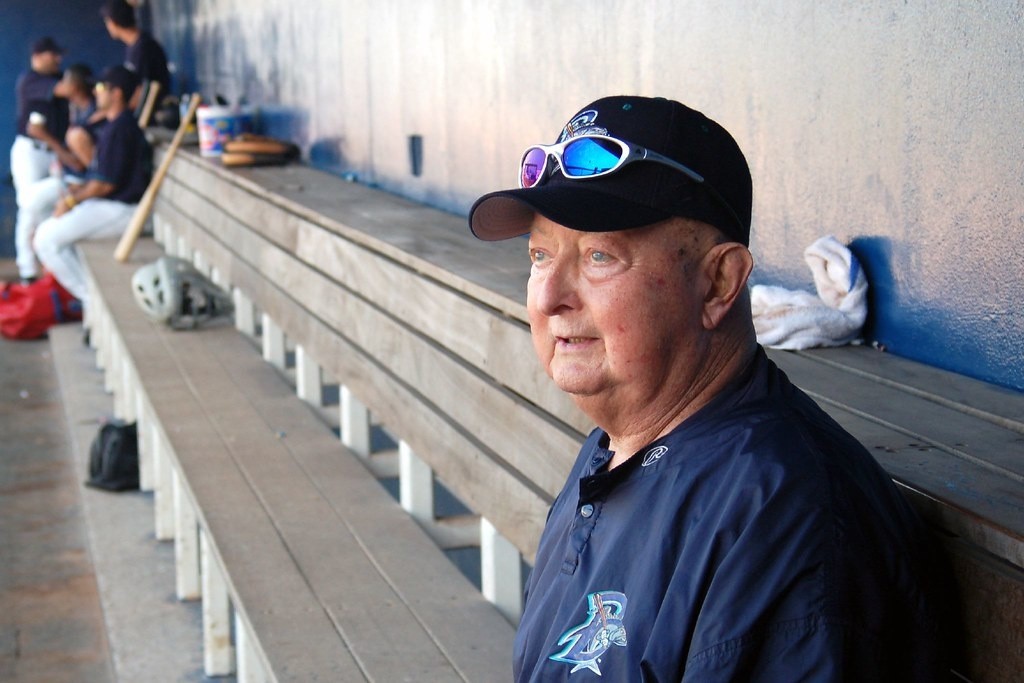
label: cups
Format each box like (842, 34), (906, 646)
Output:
(27, 111), (46, 137)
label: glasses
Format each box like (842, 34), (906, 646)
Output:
(96, 82), (111, 92)
(518, 135), (705, 191)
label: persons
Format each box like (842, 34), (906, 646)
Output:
(466, 95), (955, 682)
(9, 0), (176, 300)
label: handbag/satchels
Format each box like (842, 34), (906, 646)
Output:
(83, 424), (139, 492)
(0, 273), (83, 340)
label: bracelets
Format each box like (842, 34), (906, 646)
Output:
(66, 194), (78, 209)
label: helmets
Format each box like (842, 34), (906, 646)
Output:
(130, 253), (231, 331)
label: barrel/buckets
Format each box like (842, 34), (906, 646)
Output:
(194, 104), (259, 159)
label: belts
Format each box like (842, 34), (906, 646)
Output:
(34, 144), (52, 152)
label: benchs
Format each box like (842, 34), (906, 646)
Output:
(78, 140), (1024, 683)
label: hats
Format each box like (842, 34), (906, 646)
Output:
(32, 38), (65, 53)
(469, 96), (753, 249)
(83, 66), (136, 95)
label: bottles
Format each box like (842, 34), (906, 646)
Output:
(179, 94), (196, 135)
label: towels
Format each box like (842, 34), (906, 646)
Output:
(750, 234), (869, 352)
